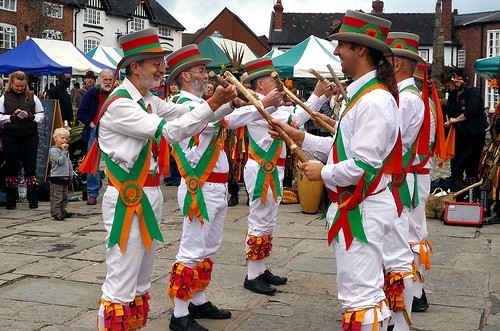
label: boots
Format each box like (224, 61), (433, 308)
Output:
(5, 186), (18, 210)
(26, 184), (39, 209)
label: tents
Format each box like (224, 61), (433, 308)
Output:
(86, 45), (124, 69)
(262, 48), (286, 58)
(0, 38), (116, 77)
(269, 35), (344, 78)
(197, 36), (261, 74)
(474, 54), (500, 79)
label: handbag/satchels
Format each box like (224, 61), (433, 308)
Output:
(425, 187), (456, 220)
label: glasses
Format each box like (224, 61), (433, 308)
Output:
(184, 69), (208, 74)
(139, 58), (166, 70)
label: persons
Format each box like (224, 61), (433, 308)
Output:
(268, 10), (402, 331)
(79, 29), (258, 331)
(311, 32), (429, 331)
(412, 59), (446, 312)
(161, 44), (293, 331)
(243, 58), (338, 295)
(0, 68), (500, 224)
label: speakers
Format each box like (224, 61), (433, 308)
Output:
(444, 201), (484, 227)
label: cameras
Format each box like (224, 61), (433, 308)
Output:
(17, 110), (28, 119)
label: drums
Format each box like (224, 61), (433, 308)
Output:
(297, 160), (323, 215)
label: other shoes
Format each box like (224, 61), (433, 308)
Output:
(165, 180), (177, 186)
(411, 288), (429, 312)
(228, 196), (238, 206)
(62, 212), (73, 218)
(87, 196), (97, 205)
(55, 214), (66, 220)
(488, 214), (500, 224)
(246, 198), (249, 206)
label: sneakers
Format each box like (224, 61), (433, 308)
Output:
(244, 274), (277, 294)
(188, 301), (231, 320)
(261, 269), (287, 285)
(169, 313), (208, 331)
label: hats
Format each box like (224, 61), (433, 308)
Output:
(165, 43), (212, 85)
(451, 69), (469, 85)
(327, 9), (393, 54)
(117, 27), (174, 70)
(80, 71), (98, 79)
(242, 56), (283, 84)
(385, 32), (426, 64)
